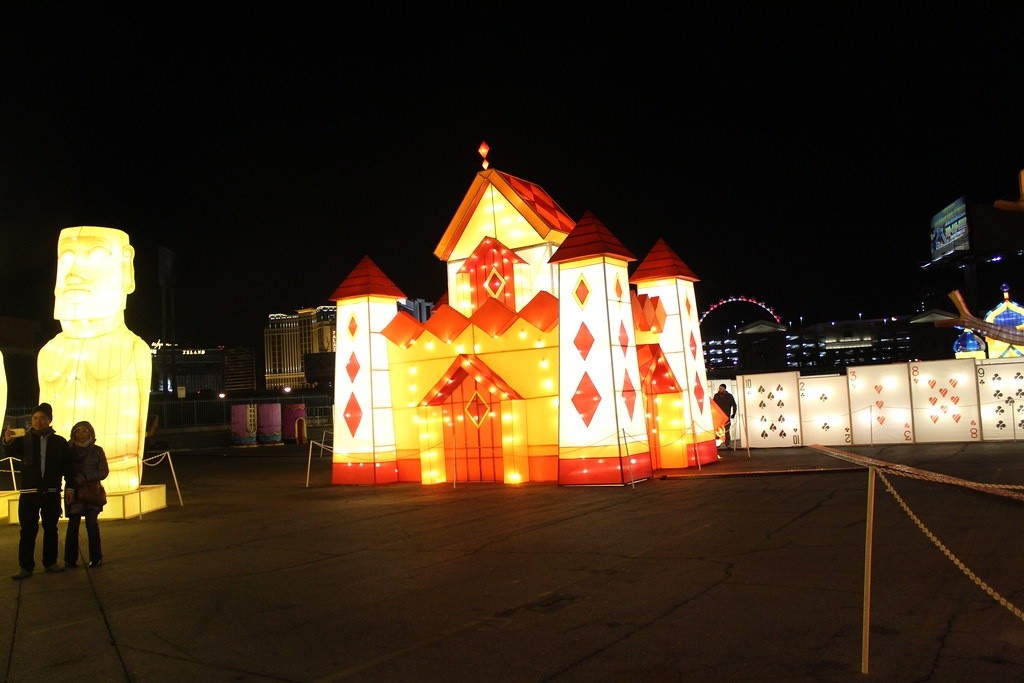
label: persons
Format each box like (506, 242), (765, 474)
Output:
(1, 403), (76, 580)
(61, 421), (108, 568)
(711, 384), (737, 450)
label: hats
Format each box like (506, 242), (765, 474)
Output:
(32, 403), (53, 418)
(720, 384), (726, 389)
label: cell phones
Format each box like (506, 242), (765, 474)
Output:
(10, 428), (26, 437)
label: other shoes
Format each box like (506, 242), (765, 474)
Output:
(44, 563), (66, 573)
(88, 555), (102, 569)
(65, 562), (78, 569)
(11, 567), (34, 580)
(726, 442), (732, 448)
